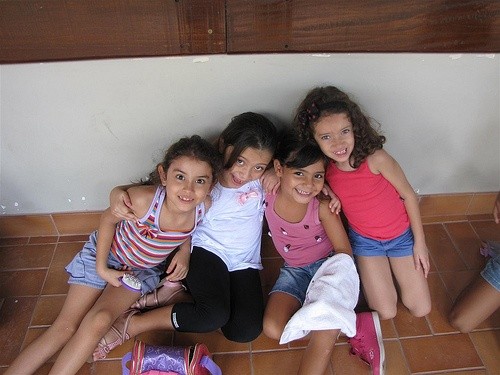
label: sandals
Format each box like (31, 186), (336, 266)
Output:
(123, 277), (187, 313)
(480, 239), (496, 258)
(87, 309), (141, 363)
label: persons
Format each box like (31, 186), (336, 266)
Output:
(0, 135), (218, 375)
(262, 138), (385, 375)
(87, 111), (342, 363)
(292, 85), (432, 321)
(447, 190), (500, 334)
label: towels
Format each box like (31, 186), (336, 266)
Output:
(278, 252), (360, 345)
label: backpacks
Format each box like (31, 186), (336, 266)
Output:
(129, 339), (222, 375)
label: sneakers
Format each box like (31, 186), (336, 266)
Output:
(344, 311), (385, 375)
